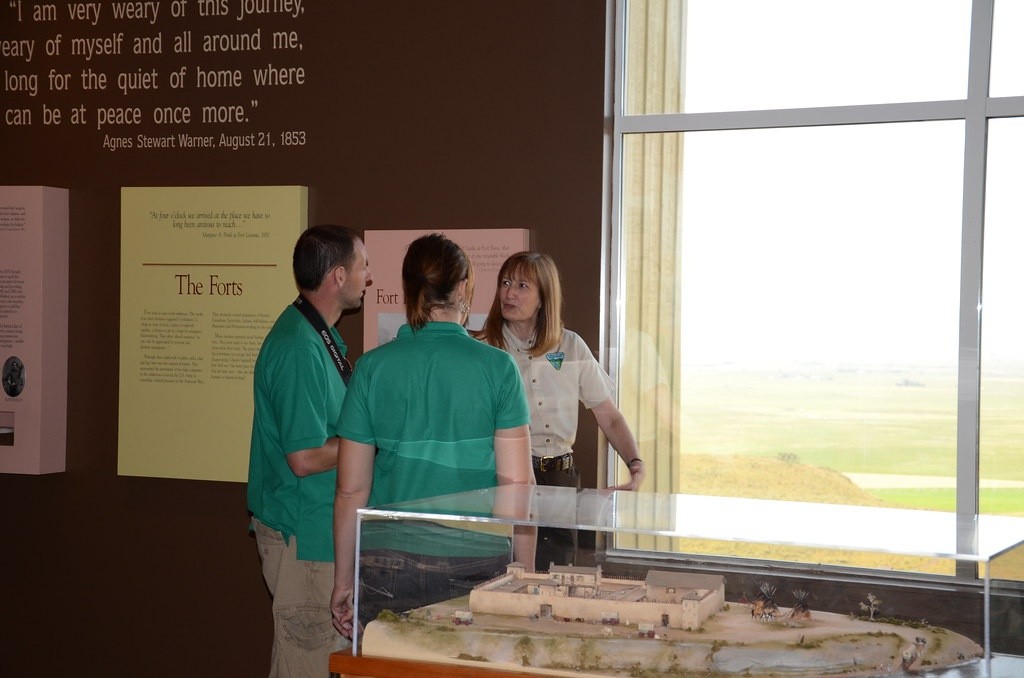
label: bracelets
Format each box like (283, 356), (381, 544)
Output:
(628, 458), (641, 468)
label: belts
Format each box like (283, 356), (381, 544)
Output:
(532, 453), (574, 473)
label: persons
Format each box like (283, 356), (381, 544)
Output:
(246, 225), (373, 678)
(332, 234), (536, 639)
(471, 250), (645, 567)
